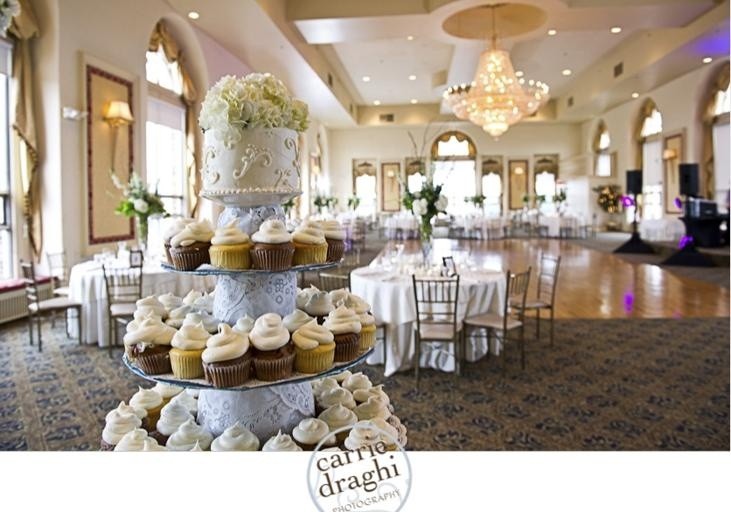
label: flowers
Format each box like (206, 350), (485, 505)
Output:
(103, 169), (172, 241)
(395, 118), (459, 239)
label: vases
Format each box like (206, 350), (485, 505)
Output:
(420, 239), (432, 268)
(136, 220), (150, 252)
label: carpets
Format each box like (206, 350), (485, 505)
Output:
(1, 314), (731, 450)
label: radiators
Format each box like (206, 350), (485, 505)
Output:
(1, 282), (58, 324)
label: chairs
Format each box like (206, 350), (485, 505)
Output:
(463, 264), (534, 377)
(45, 250), (70, 331)
(20, 259), (83, 354)
(314, 187), (599, 244)
(317, 270), (390, 369)
(412, 274), (463, 389)
(509, 251), (563, 347)
(100, 263), (145, 359)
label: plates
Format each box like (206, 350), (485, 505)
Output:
(160, 264), (344, 277)
(122, 345), (368, 392)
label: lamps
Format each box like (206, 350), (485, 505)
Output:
(663, 147), (680, 184)
(442, 4), (552, 139)
(102, 100), (135, 177)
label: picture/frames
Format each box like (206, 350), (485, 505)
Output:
(130, 249), (143, 267)
(441, 256), (458, 277)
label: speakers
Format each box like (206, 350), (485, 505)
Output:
(678, 164), (698, 195)
(625, 169), (642, 195)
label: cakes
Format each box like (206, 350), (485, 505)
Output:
(100, 73), (406, 451)
